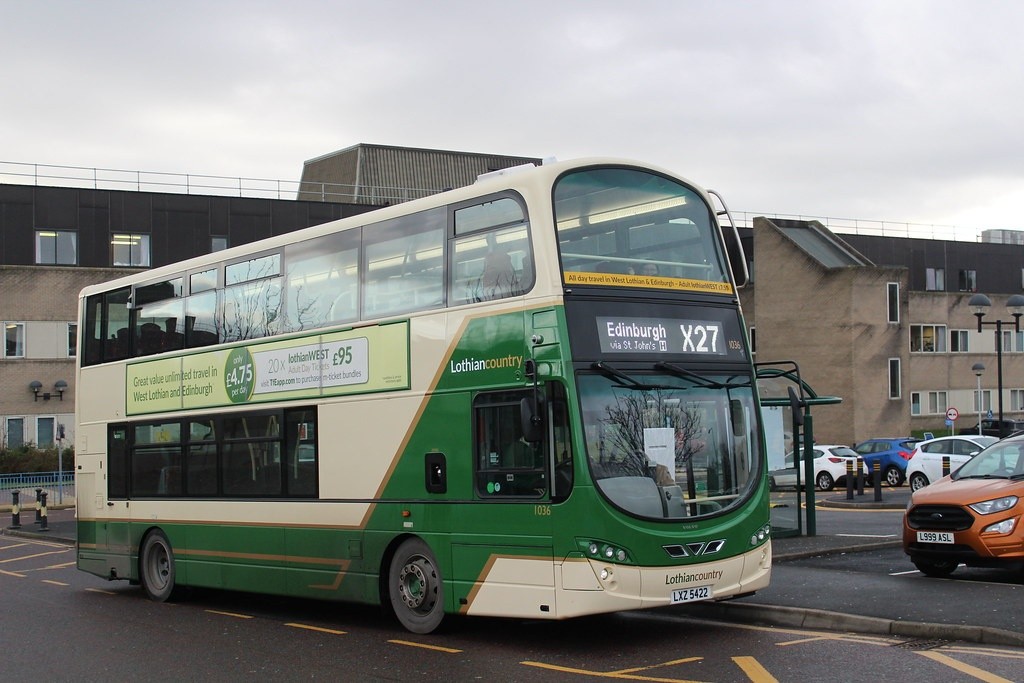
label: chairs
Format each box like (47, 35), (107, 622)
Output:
(156, 461), (183, 496)
(867, 445), (877, 453)
(879, 444), (886, 451)
(112, 246), (532, 362)
(940, 441), (963, 455)
(501, 437), (542, 490)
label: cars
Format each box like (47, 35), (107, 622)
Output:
(958, 418), (1024, 440)
(852, 437), (925, 487)
(905, 435), (1020, 492)
(768, 445), (869, 491)
(901, 431), (1024, 577)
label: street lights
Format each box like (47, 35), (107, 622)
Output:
(968, 294), (1024, 439)
(970, 363), (985, 435)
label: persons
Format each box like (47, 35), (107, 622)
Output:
(615, 257), (659, 276)
(484, 235), (515, 287)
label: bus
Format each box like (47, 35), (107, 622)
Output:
(74, 156), (773, 635)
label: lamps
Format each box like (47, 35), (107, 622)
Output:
(29, 380), (68, 401)
